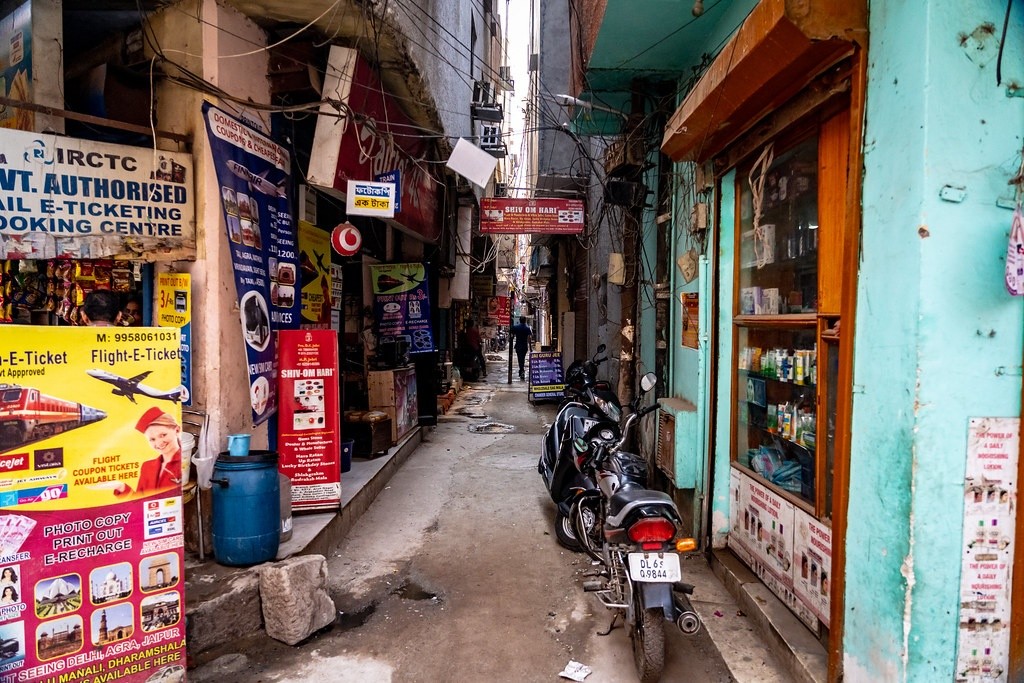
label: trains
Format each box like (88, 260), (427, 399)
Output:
(0, 383), (108, 452)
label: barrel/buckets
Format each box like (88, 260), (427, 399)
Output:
(210, 449), (280, 568)
(279, 473), (292, 543)
(227, 433), (253, 456)
(181, 432), (196, 487)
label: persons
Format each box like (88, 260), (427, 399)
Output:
(82, 289), (121, 327)
(0, 568), (19, 605)
(744, 512), (749, 530)
(488, 297), (498, 312)
(822, 572), (828, 594)
(466, 320), (487, 376)
(751, 517), (756, 535)
(802, 556), (808, 578)
(811, 565), (817, 585)
(317, 275), (331, 329)
(513, 316), (532, 380)
(758, 522), (763, 540)
(120, 289), (143, 326)
(88, 407), (181, 498)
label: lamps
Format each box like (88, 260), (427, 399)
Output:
(555, 93), (629, 123)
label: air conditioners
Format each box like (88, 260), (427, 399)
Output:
(471, 79), (502, 147)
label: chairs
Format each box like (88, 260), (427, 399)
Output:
(435, 348), (446, 394)
(181, 408), (209, 564)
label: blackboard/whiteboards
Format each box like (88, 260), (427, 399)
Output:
(530, 352), (566, 398)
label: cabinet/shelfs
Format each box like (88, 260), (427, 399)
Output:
(735, 185), (819, 277)
(735, 366), (816, 455)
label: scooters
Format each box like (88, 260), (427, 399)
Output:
(537, 380), (622, 552)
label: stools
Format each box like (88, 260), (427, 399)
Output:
(344, 417), (393, 459)
(438, 361), (455, 380)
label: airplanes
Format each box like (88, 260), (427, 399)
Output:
(85, 368), (181, 405)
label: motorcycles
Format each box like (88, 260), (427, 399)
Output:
(461, 341), (486, 382)
(567, 372), (703, 683)
(556, 342), (624, 414)
